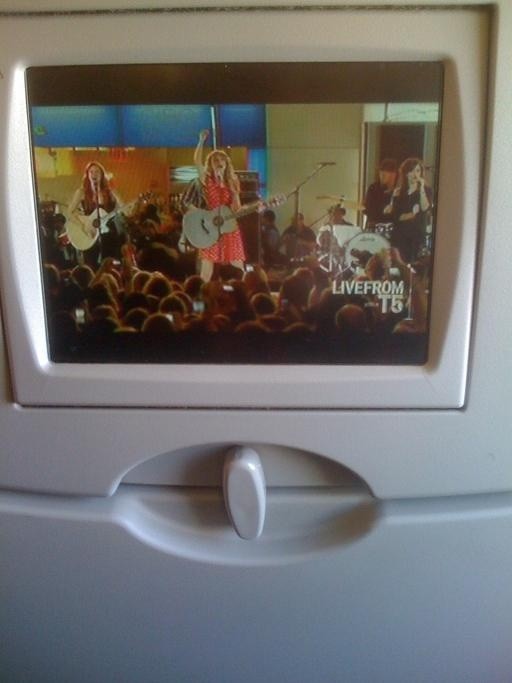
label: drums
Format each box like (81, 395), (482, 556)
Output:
(343, 233), (393, 275)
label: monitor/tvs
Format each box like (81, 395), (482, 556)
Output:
(0, 7), (490, 411)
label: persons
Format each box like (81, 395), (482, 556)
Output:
(193, 126), (267, 284)
(390, 157), (435, 266)
(36, 202), (435, 361)
(65, 160), (138, 270)
(360, 156), (400, 244)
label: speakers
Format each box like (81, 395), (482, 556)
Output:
(236, 194), (261, 266)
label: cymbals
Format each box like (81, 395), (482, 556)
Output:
(315, 193), (353, 201)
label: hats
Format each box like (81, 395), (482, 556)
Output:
(376, 159), (397, 172)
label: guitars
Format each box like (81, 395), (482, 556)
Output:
(67, 189), (154, 250)
(182, 192), (287, 248)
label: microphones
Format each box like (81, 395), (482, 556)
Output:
(92, 176), (98, 191)
(318, 161), (336, 166)
(217, 165), (223, 180)
